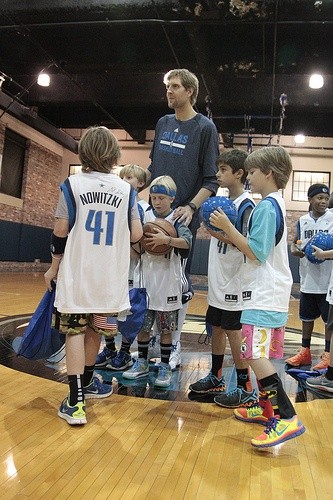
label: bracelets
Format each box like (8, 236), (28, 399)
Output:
(51, 253), (63, 260)
(167, 236), (172, 246)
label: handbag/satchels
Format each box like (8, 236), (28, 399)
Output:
(197, 322), (212, 345)
(116, 288), (149, 344)
(15, 281), (66, 361)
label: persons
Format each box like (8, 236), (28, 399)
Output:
(129, 69), (221, 372)
(284, 183), (333, 371)
(91, 165), (194, 388)
(201, 146), (306, 448)
(47, 125), (144, 425)
(306, 243), (333, 393)
(189, 150), (256, 409)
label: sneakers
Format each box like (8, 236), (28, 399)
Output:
(94, 346), (118, 366)
(233, 402), (274, 426)
(189, 372), (226, 393)
(153, 365), (171, 387)
(105, 352), (133, 370)
(122, 358), (149, 379)
(57, 391), (87, 425)
(82, 377), (113, 400)
(313, 351), (330, 371)
(153, 340), (181, 372)
(285, 346), (312, 366)
(250, 414), (306, 448)
(129, 336), (159, 362)
(306, 374), (333, 392)
(214, 387), (258, 408)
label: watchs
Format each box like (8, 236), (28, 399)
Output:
(187, 202), (197, 212)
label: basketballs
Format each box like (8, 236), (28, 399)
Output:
(310, 232), (333, 250)
(137, 203), (144, 223)
(139, 219), (178, 255)
(200, 196), (239, 231)
(305, 242), (325, 264)
